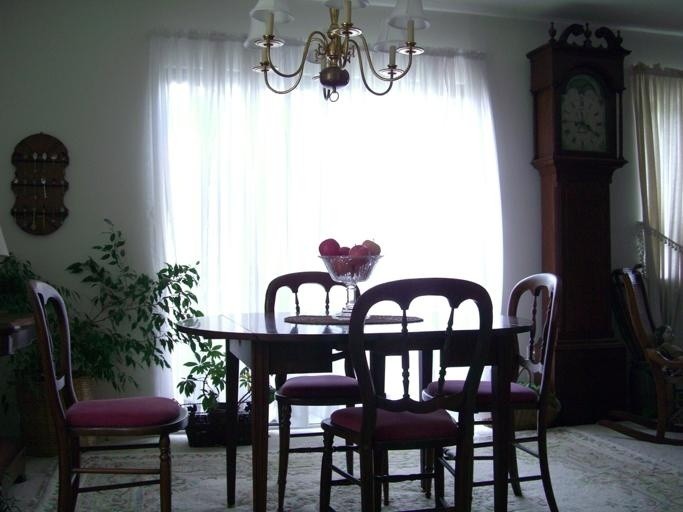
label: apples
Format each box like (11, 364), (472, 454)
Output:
(319, 238), (382, 278)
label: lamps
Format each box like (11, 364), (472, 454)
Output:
(241, 0), (433, 102)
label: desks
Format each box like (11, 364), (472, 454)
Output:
(176, 310), (533, 511)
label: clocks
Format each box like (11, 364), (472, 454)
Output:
(527, 38), (630, 171)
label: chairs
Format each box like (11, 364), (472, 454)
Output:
(603, 260), (682, 443)
(264, 270), (493, 511)
(422, 272), (561, 511)
(26, 277), (190, 511)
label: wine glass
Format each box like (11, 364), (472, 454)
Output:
(315, 255), (383, 321)
